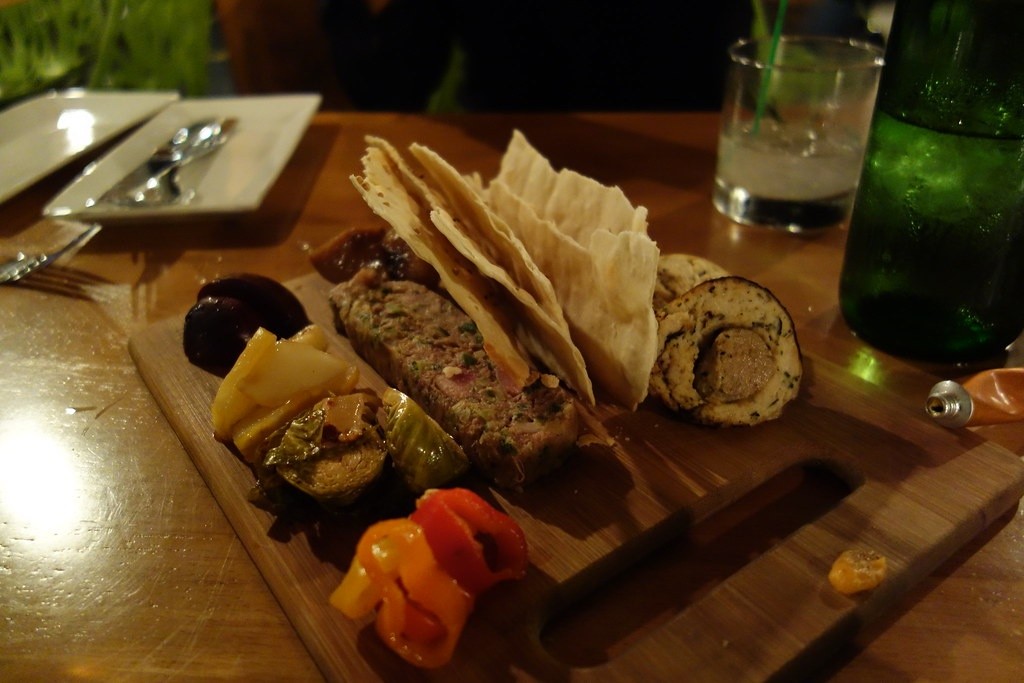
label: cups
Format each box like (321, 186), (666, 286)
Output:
(711, 34), (886, 230)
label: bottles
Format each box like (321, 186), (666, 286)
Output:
(838, 1), (1024, 360)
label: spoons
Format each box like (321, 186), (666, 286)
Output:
(119, 114), (238, 210)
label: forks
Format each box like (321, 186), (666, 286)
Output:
(0, 221), (99, 282)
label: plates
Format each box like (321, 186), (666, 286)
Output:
(42, 89), (321, 224)
(1, 87), (180, 211)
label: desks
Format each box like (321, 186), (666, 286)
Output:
(0, 102), (1024, 683)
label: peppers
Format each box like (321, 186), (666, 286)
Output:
(327, 485), (527, 669)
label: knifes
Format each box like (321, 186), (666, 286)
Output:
(90, 120), (213, 204)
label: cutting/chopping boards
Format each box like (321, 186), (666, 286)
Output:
(128, 270), (1023, 683)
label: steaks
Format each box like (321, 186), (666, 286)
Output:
(327, 265), (581, 488)
